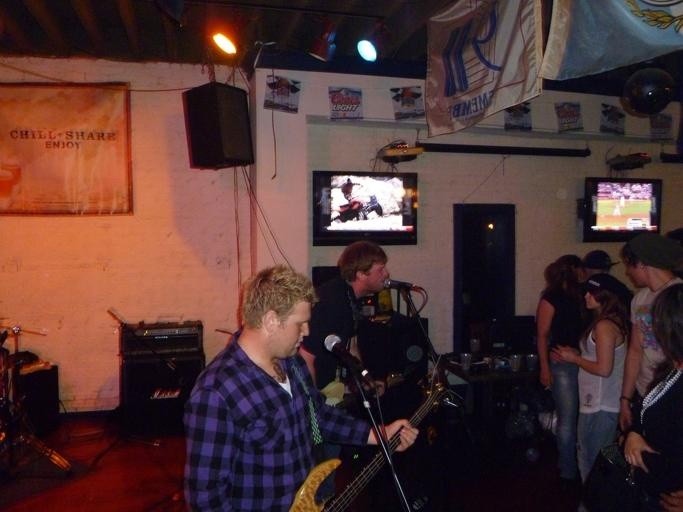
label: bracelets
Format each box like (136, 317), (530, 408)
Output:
(619, 396), (631, 402)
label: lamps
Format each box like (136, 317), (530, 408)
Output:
(356, 16), (400, 63)
(208, 3), (248, 56)
(307, 17), (341, 63)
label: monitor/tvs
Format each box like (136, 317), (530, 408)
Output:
(584, 176), (663, 242)
(313, 171), (417, 246)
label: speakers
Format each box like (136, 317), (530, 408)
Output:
(119, 356), (205, 436)
(182, 83), (255, 171)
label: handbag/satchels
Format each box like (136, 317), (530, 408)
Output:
(582, 448), (661, 511)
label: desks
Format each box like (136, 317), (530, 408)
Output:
(438, 351), (553, 433)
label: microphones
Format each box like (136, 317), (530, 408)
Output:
(383, 276), (414, 294)
(324, 334), (371, 379)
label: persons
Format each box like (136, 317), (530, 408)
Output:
(549, 273), (633, 483)
(337, 178), (364, 203)
(618, 282), (682, 495)
(583, 250), (634, 305)
(299, 241), (389, 457)
(185, 267), (420, 512)
(620, 230), (682, 431)
(537, 255), (594, 480)
(658, 491), (683, 512)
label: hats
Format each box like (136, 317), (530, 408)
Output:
(582, 249), (618, 268)
(628, 233), (683, 269)
(582, 274), (613, 292)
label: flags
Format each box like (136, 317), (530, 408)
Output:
(536, 1), (682, 81)
(424, 0), (544, 139)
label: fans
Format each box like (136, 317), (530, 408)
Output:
(618, 65), (674, 119)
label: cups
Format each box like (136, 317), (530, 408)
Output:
(527, 355), (537, 371)
(460, 354), (471, 371)
(470, 336), (480, 354)
(510, 355), (519, 371)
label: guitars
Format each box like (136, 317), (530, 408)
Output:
(318, 373), (405, 414)
(286, 384), (447, 511)
